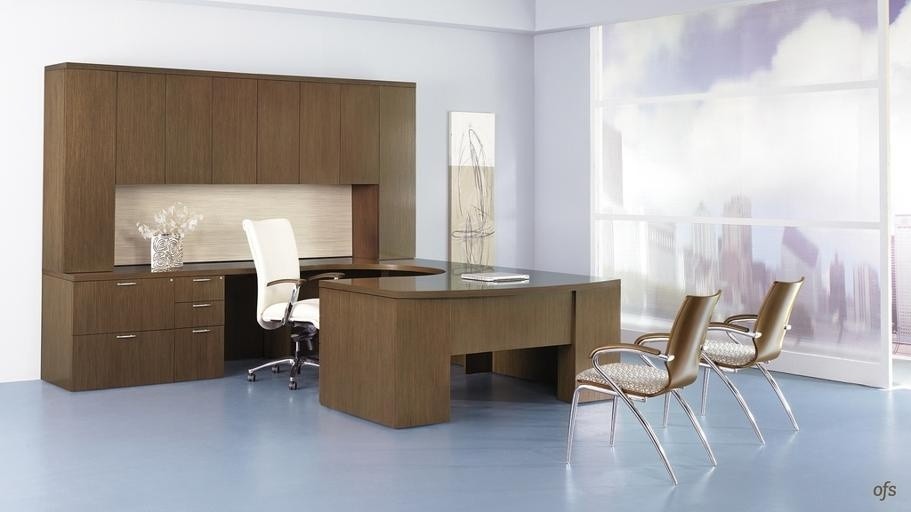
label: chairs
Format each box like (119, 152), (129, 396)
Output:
(567, 289), (724, 484)
(663, 276), (805, 442)
(242, 218), (347, 390)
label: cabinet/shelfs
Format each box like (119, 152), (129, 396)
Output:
(41, 258), (621, 429)
(42, 62), (416, 274)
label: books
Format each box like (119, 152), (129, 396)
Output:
(463, 279), (530, 285)
(462, 271), (530, 281)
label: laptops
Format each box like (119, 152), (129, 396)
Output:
(461, 273), (529, 281)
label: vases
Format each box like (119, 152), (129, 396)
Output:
(151, 236), (172, 273)
(159, 233), (185, 268)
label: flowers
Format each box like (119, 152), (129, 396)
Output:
(136, 201), (204, 240)
